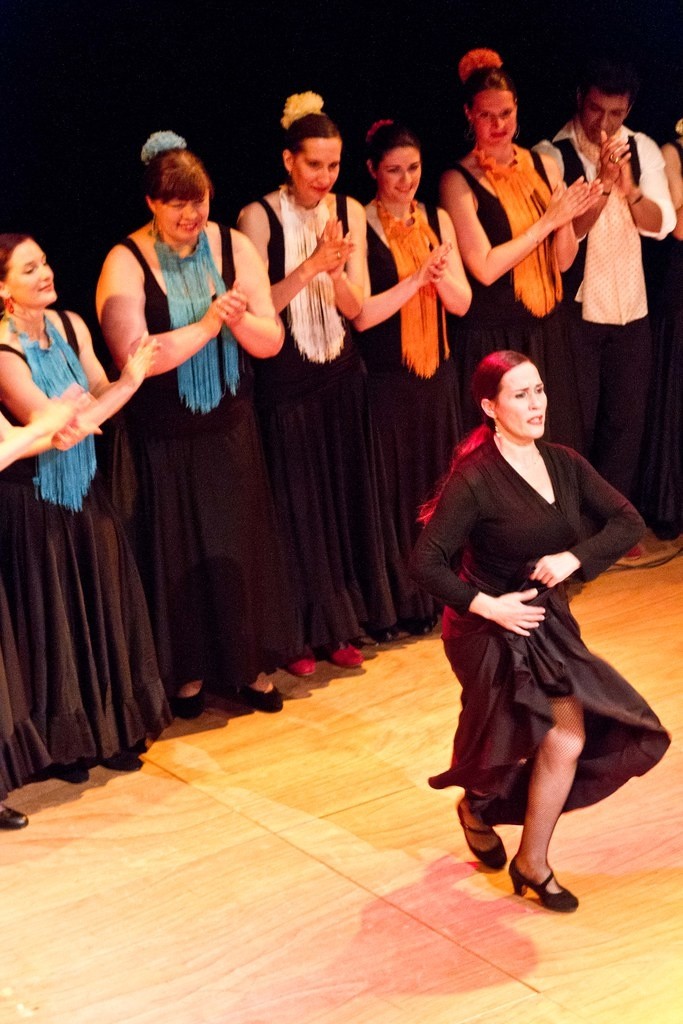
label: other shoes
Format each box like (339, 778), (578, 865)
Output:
(49, 756), (89, 783)
(411, 621), (430, 634)
(171, 680), (204, 718)
(241, 685), (283, 712)
(287, 657), (316, 676)
(329, 642), (364, 667)
(0, 802), (28, 828)
(426, 615), (437, 627)
(96, 749), (138, 771)
(380, 630), (393, 641)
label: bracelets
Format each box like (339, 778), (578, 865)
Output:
(523, 230), (539, 245)
(602, 190), (611, 195)
(626, 189), (642, 204)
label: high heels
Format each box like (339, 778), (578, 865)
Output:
(510, 860), (578, 912)
(457, 803), (507, 868)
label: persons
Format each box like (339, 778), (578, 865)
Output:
(405, 350), (671, 911)
(95, 131), (285, 720)
(0, 236), (160, 830)
(362, 119), (472, 633)
(440, 48), (683, 541)
(236, 92), (367, 676)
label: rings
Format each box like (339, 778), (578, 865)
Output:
(337, 251), (341, 259)
(609, 154), (618, 163)
